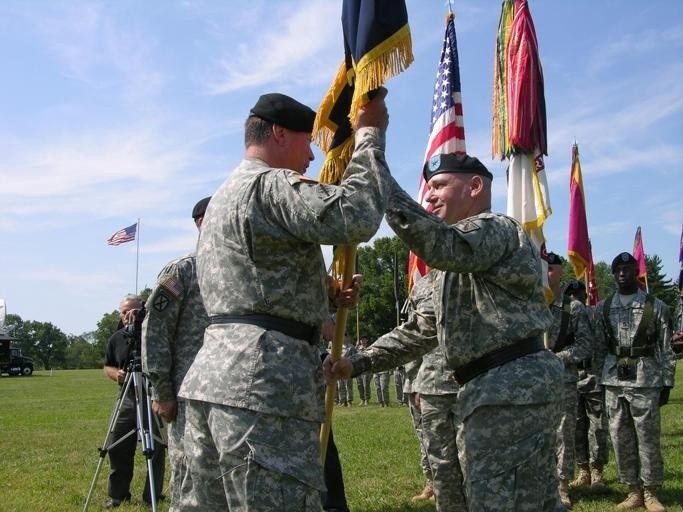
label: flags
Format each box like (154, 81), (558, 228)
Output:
(107, 222), (136, 246)
(312, 0), (682, 317)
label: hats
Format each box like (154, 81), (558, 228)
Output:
(192, 197), (211, 218)
(423, 153), (493, 183)
(249, 94), (316, 133)
(565, 282), (585, 294)
(612, 253), (637, 266)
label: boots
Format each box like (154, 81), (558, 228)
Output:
(413, 479), (435, 500)
(558, 469), (604, 506)
(617, 484), (665, 512)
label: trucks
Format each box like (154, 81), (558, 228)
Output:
(0, 333), (33, 378)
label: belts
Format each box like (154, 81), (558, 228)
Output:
(209, 313), (321, 346)
(451, 337), (545, 385)
(611, 346), (653, 358)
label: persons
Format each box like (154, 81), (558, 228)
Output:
(142, 92), (388, 512)
(103, 293), (170, 509)
(323, 150), (683, 512)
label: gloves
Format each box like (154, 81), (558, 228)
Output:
(660, 386), (669, 406)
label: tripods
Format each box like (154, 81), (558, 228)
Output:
(82, 356), (168, 512)
